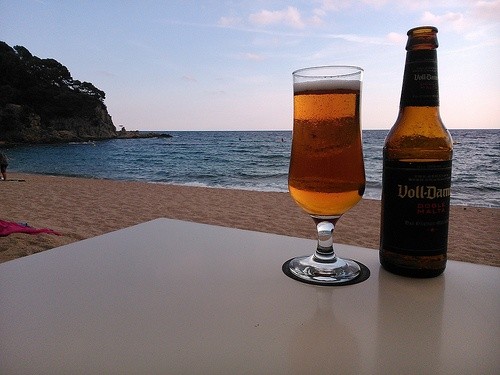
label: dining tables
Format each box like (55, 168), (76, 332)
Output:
(0, 215), (500, 375)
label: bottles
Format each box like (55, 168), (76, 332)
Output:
(379, 26), (453, 278)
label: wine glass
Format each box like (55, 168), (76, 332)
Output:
(288, 65), (366, 283)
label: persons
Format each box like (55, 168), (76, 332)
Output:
(0, 149), (9, 182)
